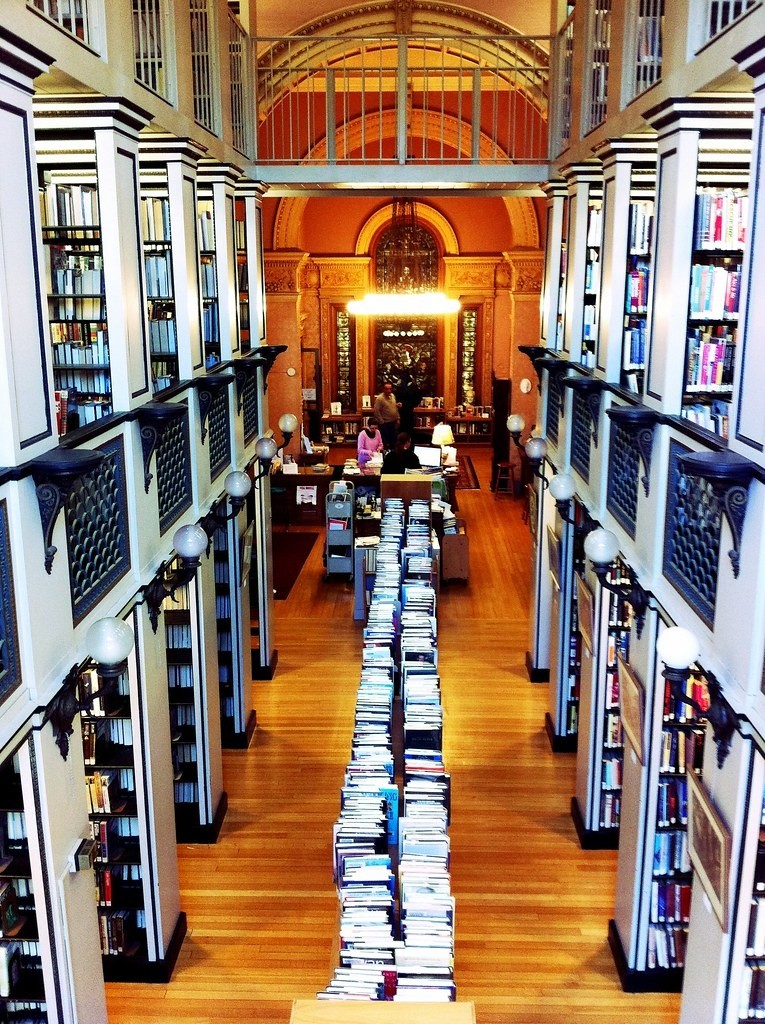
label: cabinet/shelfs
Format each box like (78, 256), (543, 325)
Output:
(640, 96), (756, 442)
(442, 519), (469, 590)
(538, 178), (569, 353)
(589, 137), (660, 401)
(77, 597), (188, 983)
(162, 536), (229, 844)
(727, 36), (765, 472)
(324, 480), (355, 584)
(0, 726), (108, 1024)
(320, 396), (493, 449)
(195, 162), (245, 372)
(570, 555), (637, 852)
(249, 439), (279, 680)
(234, 180), (271, 356)
(544, 495), (604, 754)
(677, 703), (765, 1024)
(30, 97), (155, 438)
(0, 25), (61, 469)
(209, 494), (257, 749)
(607, 597), (707, 993)
(558, 162), (603, 371)
(135, 137), (209, 398)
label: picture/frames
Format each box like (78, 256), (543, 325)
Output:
(240, 520), (255, 586)
(618, 650), (647, 765)
(685, 763), (733, 936)
(526, 484), (539, 549)
(575, 570), (594, 655)
(546, 526), (562, 593)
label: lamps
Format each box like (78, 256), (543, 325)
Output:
(251, 437), (278, 489)
(42, 617), (134, 763)
(142, 524), (209, 636)
(656, 626), (741, 772)
(432, 425), (455, 466)
(201, 470), (252, 560)
(549, 474), (597, 555)
(506, 415), (533, 454)
(270, 413), (297, 459)
(584, 530), (652, 641)
(525, 437), (558, 491)
(346, 199), (462, 337)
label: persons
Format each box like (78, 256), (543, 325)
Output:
(358, 417), (384, 468)
(464, 390), (477, 407)
(379, 432), (423, 474)
(373, 382), (402, 451)
(397, 373), (422, 439)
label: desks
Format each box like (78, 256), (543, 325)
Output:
(342, 462), (459, 515)
(271, 466), (334, 524)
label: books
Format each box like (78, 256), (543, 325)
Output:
(0, 525), (236, 1024)
(565, 528), (765, 1023)
(321, 416), (370, 443)
(414, 397), (495, 434)
(554, 183), (751, 444)
(38, 182), (250, 435)
(317, 486), (462, 1002)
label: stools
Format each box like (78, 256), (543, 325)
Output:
(494, 462), (517, 501)
